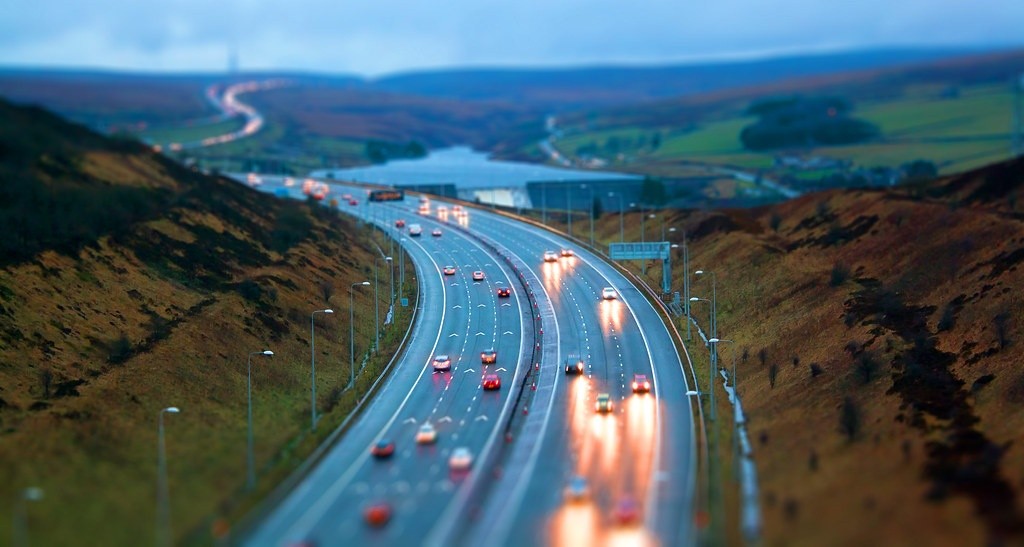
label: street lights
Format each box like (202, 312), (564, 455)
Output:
(311, 308), (334, 434)
(607, 190), (624, 243)
(374, 256), (393, 354)
(649, 213), (666, 242)
(628, 202), (646, 275)
(688, 297), (715, 421)
(695, 269), (719, 377)
(671, 242), (693, 342)
(247, 349), (275, 502)
(350, 282), (371, 389)
(709, 338), (737, 415)
(580, 184), (594, 246)
(159, 407), (181, 547)
(669, 227), (687, 314)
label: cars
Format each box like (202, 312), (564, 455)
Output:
(228, 169), (468, 237)
(595, 393), (612, 413)
(449, 447), (472, 471)
(472, 271), (484, 281)
(432, 355), (451, 373)
(479, 349), (497, 364)
(544, 246), (574, 262)
(498, 287), (510, 298)
(632, 375), (651, 393)
(563, 356), (584, 375)
(371, 437), (393, 457)
(483, 374), (502, 391)
(413, 424), (439, 444)
(602, 287), (617, 299)
(442, 266), (456, 275)
